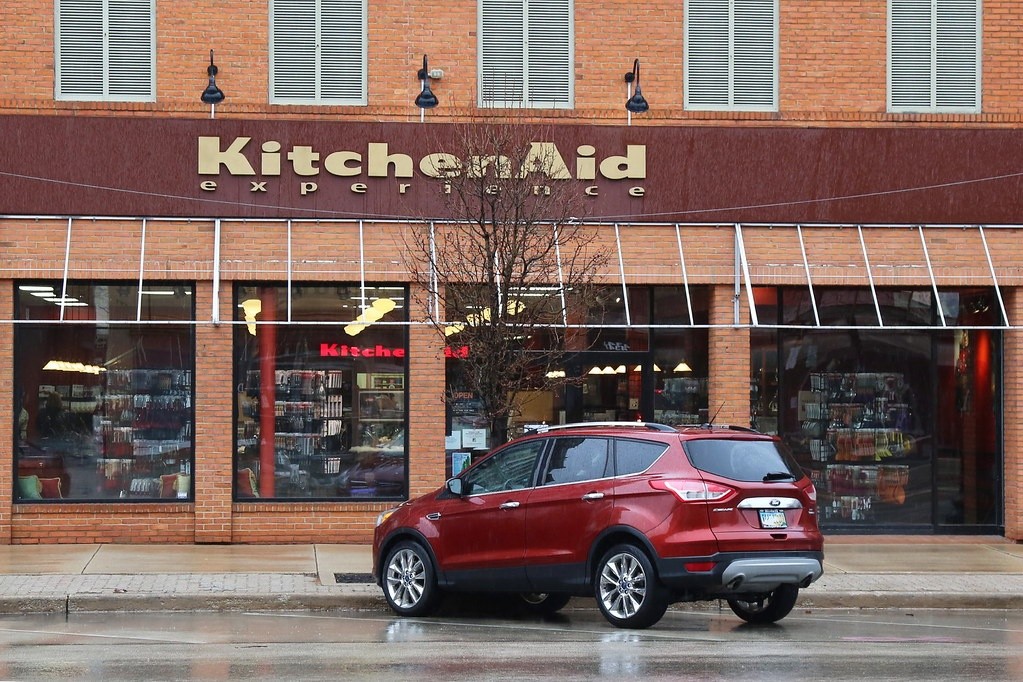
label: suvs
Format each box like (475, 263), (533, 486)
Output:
(336, 409), (505, 499)
(371, 418), (824, 628)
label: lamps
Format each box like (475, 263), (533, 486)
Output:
(200, 48), (226, 106)
(415, 54), (439, 110)
(626, 59), (650, 112)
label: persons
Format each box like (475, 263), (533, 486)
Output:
(34, 391), (75, 438)
(16, 395), (29, 442)
